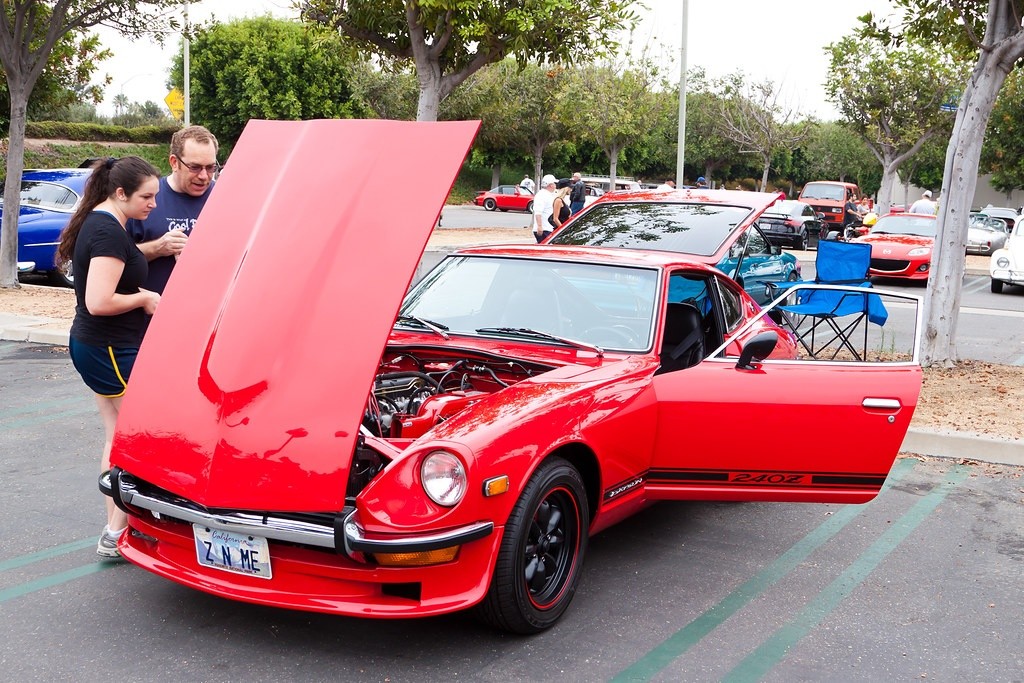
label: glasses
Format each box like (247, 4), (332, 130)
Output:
(171, 152), (220, 173)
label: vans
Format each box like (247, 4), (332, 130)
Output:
(797, 180), (862, 232)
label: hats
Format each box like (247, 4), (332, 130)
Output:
(541, 174), (559, 187)
(922, 190), (932, 198)
(558, 178), (576, 189)
(695, 177), (705, 184)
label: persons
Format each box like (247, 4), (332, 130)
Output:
(695, 177), (709, 190)
(908, 190), (936, 215)
(843, 192), (871, 234)
(771, 188), (787, 200)
(519, 174), (535, 195)
(125, 126), (220, 299)
(719, 184), (726, 190)
(53, 157), (162, 558)
(656, 178), (677, 190)
(735, 185), (743, 190)
(570, 172), (585, 217)
(532, 174), (575, 246)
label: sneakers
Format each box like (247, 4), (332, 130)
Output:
(96, 531), (121, 557)
(102, 525), (109, 536)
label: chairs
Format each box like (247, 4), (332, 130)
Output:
(654, 302), (705, 376)
(754, 238), (888, 362)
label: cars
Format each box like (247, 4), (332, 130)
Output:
(967, 207), (1019, 256)
(97, 118), (923, 634)
(563, 178), (642, 209)
(753, 200), (830, 251)
(472, 185), (535, 214)
(990, 215), (1024, 294)
(849, 213), (937, 280)
(0, 159), (106, 288)
(522, 222), (804, 324)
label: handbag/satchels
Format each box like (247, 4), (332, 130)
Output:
(548, 214), (557, 228)
